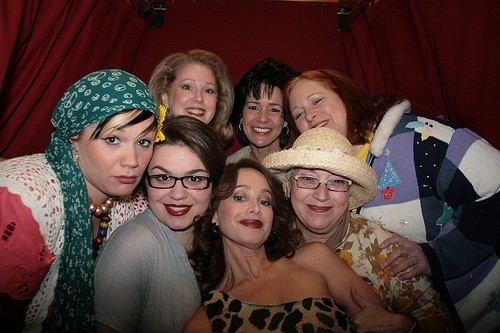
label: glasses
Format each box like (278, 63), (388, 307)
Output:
(290, 175), (353, 192)
(146, 168), (215, 190)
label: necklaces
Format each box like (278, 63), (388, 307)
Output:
(93, 215), (110, 258)
(87, 197), (114, 215)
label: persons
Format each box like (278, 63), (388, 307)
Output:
(262, 126), (443, 333)
(94, 116), (227, 333)
(0, 69), (167, 333)
(149, 50), (234, 139)
(185, 158), (389, 333)
(285, 67), (500, 333)
(230, 58), (299, 165)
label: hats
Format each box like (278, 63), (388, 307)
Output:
(261, 127), (378, 211)
